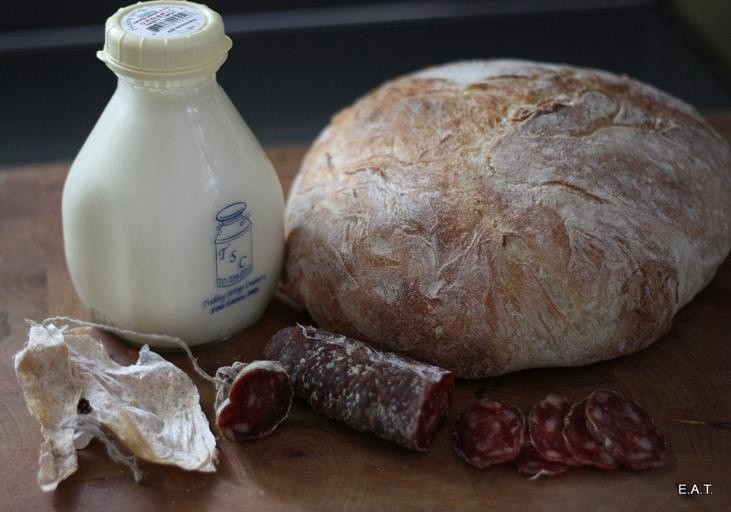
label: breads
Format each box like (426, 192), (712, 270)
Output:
(279, 56), (731, 385)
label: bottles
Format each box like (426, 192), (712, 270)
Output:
(60, 1), (286, 353)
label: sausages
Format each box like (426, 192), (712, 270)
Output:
(212, 323), (669, 475)
